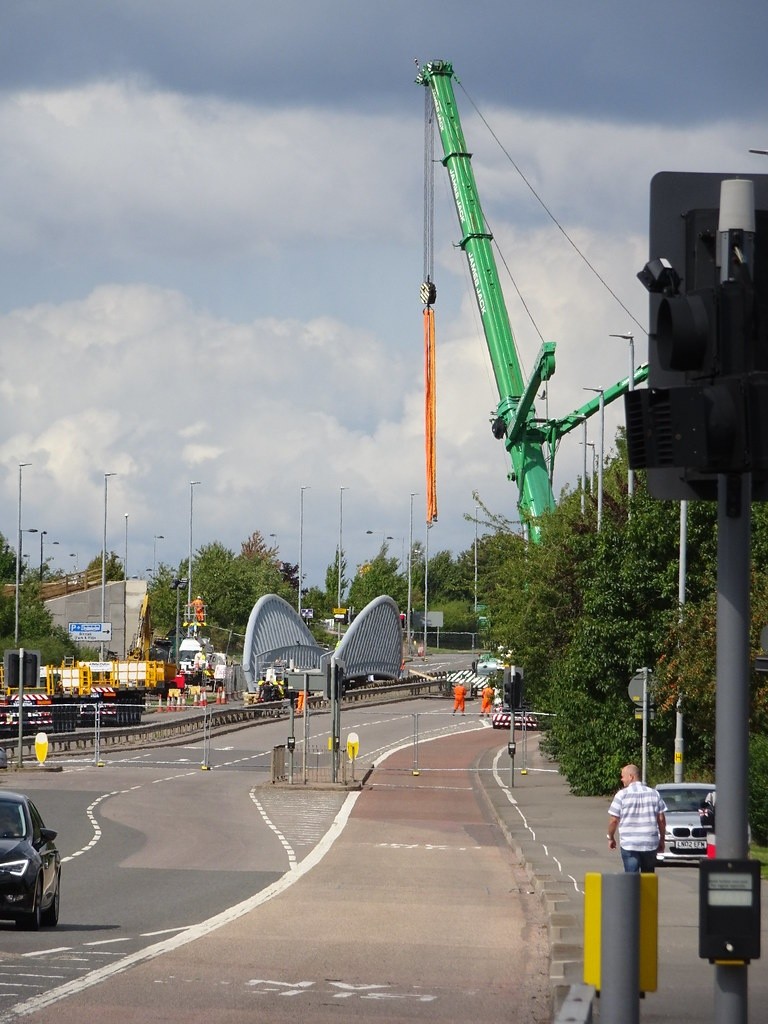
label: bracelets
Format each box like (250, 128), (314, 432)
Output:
(607, 835), (614, 840)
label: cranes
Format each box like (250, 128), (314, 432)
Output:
(412, 58), (648, 551)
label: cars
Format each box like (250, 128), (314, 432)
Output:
(0, 791), (61, 930)
(652, 782), (753, 867)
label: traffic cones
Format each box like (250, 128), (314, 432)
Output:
(167, 687), (227, 713)
(156, 693), (165, 713)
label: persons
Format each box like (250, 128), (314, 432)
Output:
(257, 676), (284, 702)
(452, 681), (466, 716)
(479, 685), (494, 717)
(607, 764), (668, 873)
(188, 596), (204, 621)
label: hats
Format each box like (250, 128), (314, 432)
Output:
(459, 681), (463, 684)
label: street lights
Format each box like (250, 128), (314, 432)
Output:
(187, 481), (202, 620)
(474, 506), (482, 613)
(568, 415), (587, 518)
(98, 472), (118, 661)
(298, 486), (312, 616)
(153, 536), (164, 578)
(424, 524), (434, 656)
(337, 487), (350, 641)
(581, 387), (604, 533)
(609, 333), (634, 522)
(15, 463), (48, 649)
(407, 492), (419, 647)
(579, 442), (595, 499)
(124, 513), (130, 580)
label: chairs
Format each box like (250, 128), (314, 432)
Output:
(663, 798), (675, 804)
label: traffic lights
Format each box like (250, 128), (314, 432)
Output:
(655, 288), (738, 476)
(504, 678), (511, 707)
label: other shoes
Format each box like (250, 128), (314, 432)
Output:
(487, 715), (489, 717)
(480, 714), (484, 717)
(461, 714), (465, 716)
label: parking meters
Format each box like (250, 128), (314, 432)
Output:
(697, 857), (760, 1024)
(287, 737), (296, 785)
